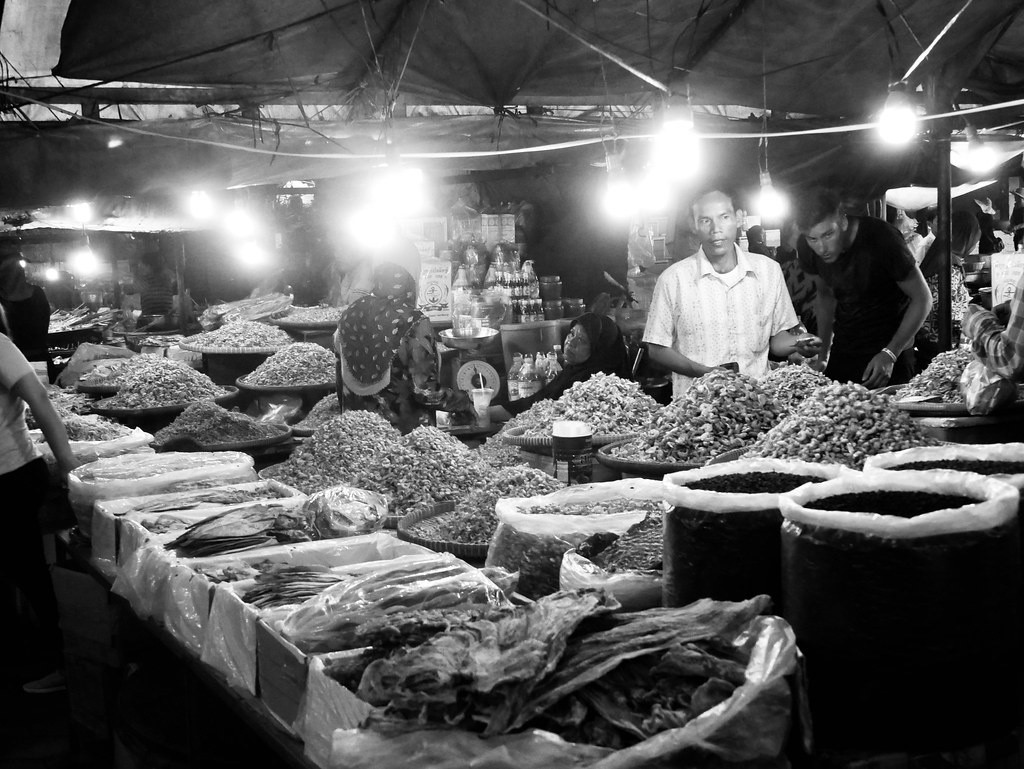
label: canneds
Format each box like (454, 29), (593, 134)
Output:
(552, 420), (594, 485)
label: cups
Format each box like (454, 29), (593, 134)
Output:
(459, 314), (490, 338)
(471, 388), (494, 427)
(653, 237), (665, 261)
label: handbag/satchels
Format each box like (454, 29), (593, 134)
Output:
(993, 238), (1005, 251)
(959, 358), (1018, 414)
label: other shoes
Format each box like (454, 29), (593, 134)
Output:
(25, 669), (61, 693)
(55, 525), (94, 567)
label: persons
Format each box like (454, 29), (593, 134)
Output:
(790, 187), (935, 389)
(322, 253), (374, 306)
(961, 270), (1024, 387)
(885, 186), (1024, 344)
(776, 219), (822, 336)
(484, 311), (633, 420)
(749, 223), (771, 257)
(131, 253), (175, 328)
(0, 303), (84, 696)
(0, 254), (56, 381)
(638, 184), (820, 403)
(329, 261), (473, 433)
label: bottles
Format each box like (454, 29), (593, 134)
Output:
(494, 269), (546, 324)
(506, 344), (566, 402)
(788, 315), (808, 368)
(622, 335), (639, 355)
(453, 264), (513, 328)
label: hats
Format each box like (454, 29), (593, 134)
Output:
(974, 197), (996, 215)
(1009, 187), (1024, 199)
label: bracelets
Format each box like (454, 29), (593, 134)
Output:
(881, 348), (897, 362)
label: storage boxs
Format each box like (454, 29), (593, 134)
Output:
(93, 478), (538, 769)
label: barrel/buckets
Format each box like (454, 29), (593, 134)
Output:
(500, 318), (562, 376)
(978, 287), (992, 308)
(551, 424), (598, 488)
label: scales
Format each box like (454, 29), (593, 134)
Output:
(438, 326), (501, 408)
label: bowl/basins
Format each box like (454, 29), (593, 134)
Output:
(140, 314), (169, 332)
